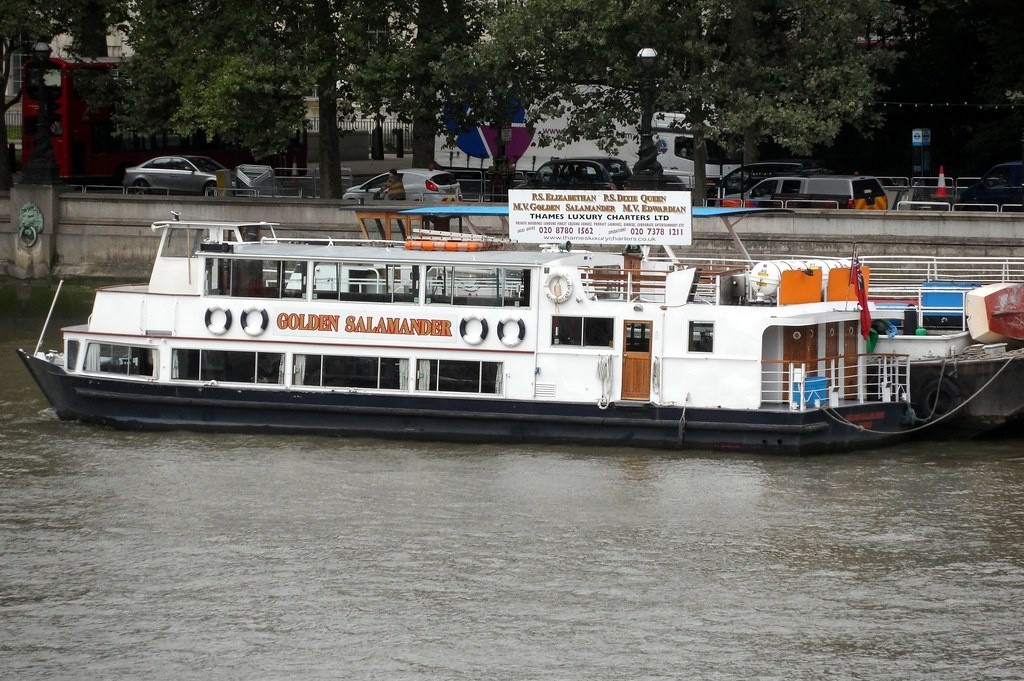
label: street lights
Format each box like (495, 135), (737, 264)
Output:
(31, 39), (56, 185)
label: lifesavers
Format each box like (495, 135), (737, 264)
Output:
(204, 271), (574, 348)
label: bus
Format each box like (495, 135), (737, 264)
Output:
(17, 52), (309, 186)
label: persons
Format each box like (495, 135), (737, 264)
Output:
(565, 164), (576, 184)
(378, 169), (406, 200)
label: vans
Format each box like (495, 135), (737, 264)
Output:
(721, 177), (887, 212)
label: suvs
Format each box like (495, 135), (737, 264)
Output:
(701, 161), (824, 210)
(514, 157), (631, 192)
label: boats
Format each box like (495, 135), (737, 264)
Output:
(15, 193), (1023, 451)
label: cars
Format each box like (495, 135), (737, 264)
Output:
(343, 166), (463, 203)
(957, 163), (1024, 211)
(122, 154), (239, 198)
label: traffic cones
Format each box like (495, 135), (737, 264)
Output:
(936, 166), (946, 200)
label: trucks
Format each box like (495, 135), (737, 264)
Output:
(438, 83), (742, 188)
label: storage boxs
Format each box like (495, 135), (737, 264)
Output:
(873, 280), (981, 335)
(793, 376), (828, 407)
